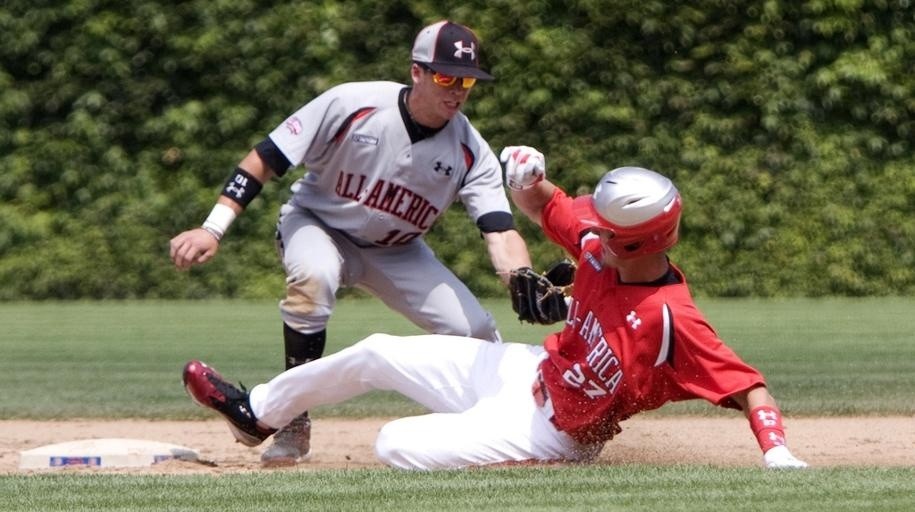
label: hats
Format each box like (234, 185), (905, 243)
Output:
(408, 18), (497, 82)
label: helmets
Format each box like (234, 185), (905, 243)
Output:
(569, 165), (685, 262)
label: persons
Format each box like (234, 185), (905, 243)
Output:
(169, 21), (576, 467)
(183, 146), (808, 473)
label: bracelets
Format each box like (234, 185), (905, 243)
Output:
(749, 406), (785, 454)
(201, 202), (236, 240)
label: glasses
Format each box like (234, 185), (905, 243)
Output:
(418, 63), (479, 89)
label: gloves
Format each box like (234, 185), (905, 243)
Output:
(499, 145), (546, 191)
(763, 444), (810, 468)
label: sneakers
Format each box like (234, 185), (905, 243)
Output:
(259, 414), (314, 468)
(180, 359), (278, 449)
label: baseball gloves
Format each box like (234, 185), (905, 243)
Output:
(508, 259), (576, 325)
